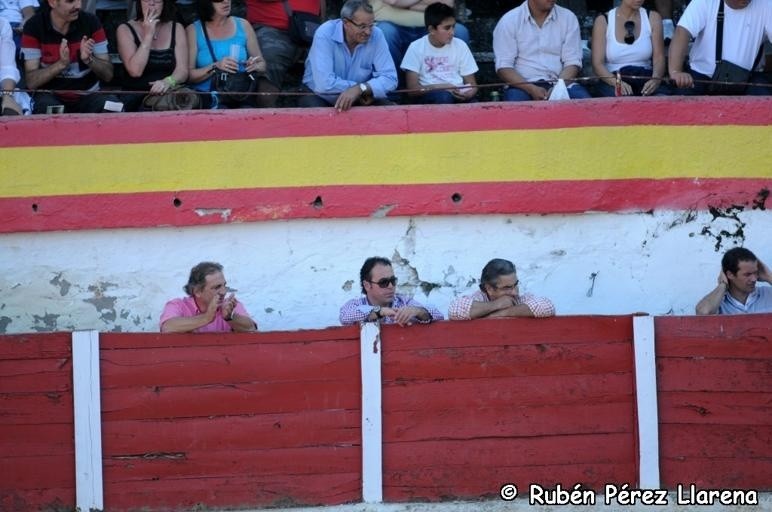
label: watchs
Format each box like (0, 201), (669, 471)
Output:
(224, 309), (238, 325)
(369, 305), (382, 318)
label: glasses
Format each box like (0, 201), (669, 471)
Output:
(344, 17), (376, 30)
(368, 276), (399, 289)
(624, 21), (636, 45)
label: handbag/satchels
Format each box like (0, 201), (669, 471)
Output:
(211, 67), (260, 106)
(139, 83), (201, 111)
(709, 60), (757, 96)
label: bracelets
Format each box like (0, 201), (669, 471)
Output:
(720, 279), (731, 288)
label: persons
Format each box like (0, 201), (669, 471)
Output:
(697, 247), (771, 317)
(1, 0), (771, 116)
(340, 255), (442, 326)
(157, 262), (256, 334)
(447, 259), (554, 317)
